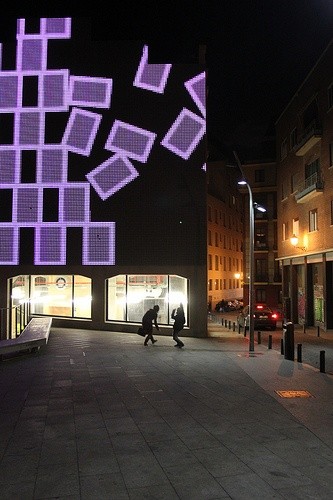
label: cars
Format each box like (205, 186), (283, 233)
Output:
(216, 299), (243, 312)
(236, 303), (276, 330)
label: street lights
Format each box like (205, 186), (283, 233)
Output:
(237, 181), (266, 352)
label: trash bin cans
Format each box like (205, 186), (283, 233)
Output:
(282, 322), (294, 361)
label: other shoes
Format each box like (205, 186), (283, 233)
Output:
(144, 343), (147, 345)
(152, 339), (157, 344)
(175, 343), (184, 348)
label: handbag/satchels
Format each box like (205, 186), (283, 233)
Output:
(138, 327), (146, 336)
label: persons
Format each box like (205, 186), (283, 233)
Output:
(171, 302), (185, 349)
(141, 304), (160, 346)
